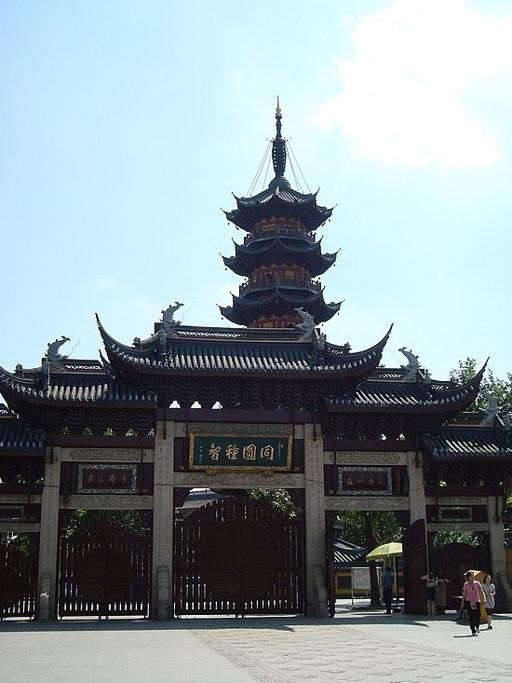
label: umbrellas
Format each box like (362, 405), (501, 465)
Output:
(365, 542), (402, 588)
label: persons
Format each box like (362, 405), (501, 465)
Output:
(460, 571), (487, 636)
(420, 571), (439, 618)
(382, 566), (394, 614)
(481, 573), (496, 628)
